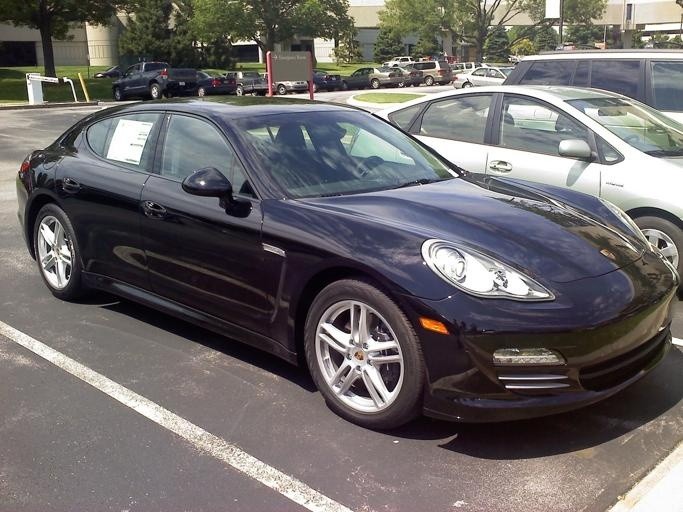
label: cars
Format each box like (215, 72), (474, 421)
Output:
(93, 66), (122, 79)
(563, 39), (573, 46)
(15, 96), (678, 432)
(347, 85), (682, 295)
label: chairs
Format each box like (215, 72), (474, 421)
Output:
(272, 124), (329, 188)
(550, 106), (593, 156)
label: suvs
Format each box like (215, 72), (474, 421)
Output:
(485, 49), (682, 129)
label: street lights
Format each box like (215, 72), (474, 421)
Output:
(601, 25), (613, 50)
(618, 30), (625, 41)
